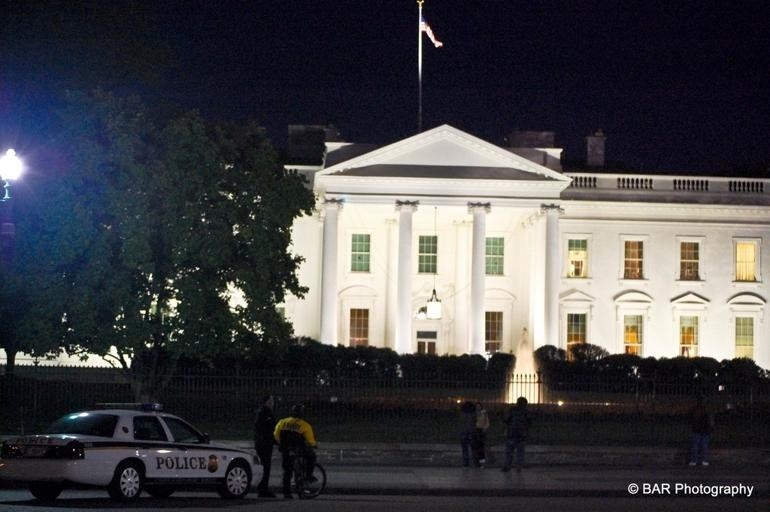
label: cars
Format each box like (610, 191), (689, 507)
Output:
(0, 402), (265, 502)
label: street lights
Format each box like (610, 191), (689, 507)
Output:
(0, 147), (22, 251)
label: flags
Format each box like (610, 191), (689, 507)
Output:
(421, 14), (445, 50)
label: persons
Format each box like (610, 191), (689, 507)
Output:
(474, 401), (492, 464)
(501, 396), (533, 475)
(686, 397), (714, 470)
(253, 393), (279, 501)
(457, 399), (487, 472)
(272, 402), (320, 502)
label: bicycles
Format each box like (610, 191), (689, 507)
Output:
(289, 447), (327, 501)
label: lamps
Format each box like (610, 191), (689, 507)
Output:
(426, 204), (442, 320)
